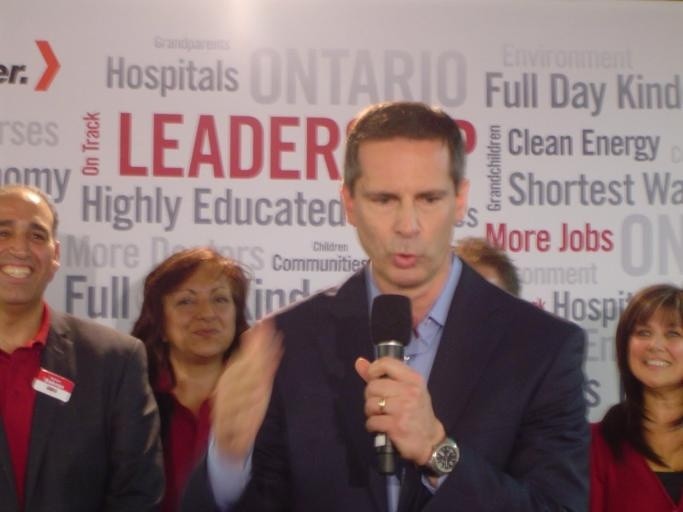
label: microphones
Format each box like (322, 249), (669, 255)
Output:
(370, 294), (413, 473)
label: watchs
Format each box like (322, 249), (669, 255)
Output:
(410, 436), (461, 478)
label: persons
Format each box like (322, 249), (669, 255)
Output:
(449, 233), (519, 299)
(583, 284), (683, 512)
(175, 101), (592, 510)
(0, 183), (166, 510)
(129, 246), (250, 510)
